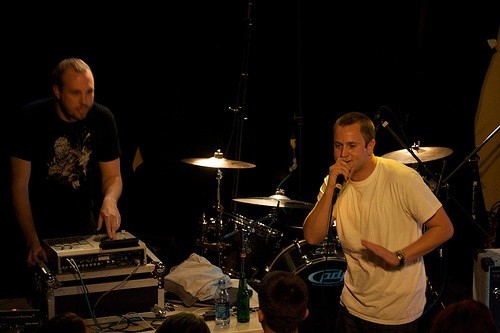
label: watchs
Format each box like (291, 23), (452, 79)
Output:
(395, 251), (405, 270)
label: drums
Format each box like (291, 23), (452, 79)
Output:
(267, 236), (347, 333)
(201, 210), (284, 286)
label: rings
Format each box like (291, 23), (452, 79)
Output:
(115, 222), (121, 226)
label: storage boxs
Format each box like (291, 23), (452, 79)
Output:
(37, 247), (166, 325)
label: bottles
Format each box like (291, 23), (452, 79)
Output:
(236, 273), (251, 323)
(214, 279), (231, 328)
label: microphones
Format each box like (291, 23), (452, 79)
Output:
(332, 174), (344, 205)
(374, 111), (381, 132)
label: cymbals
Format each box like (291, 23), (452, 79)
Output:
(181, 158), (256, 169)
(233, 194), (315, 209)
(379, 147), (453, 165)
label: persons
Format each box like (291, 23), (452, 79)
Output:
(154, 312), (211, 333)
(41, 313), (86, 333)
(256, 269), (308, 333)
(432, 300), (497, 333)
(7, 58), (124, 268)
(302, 111), (455, 333)
